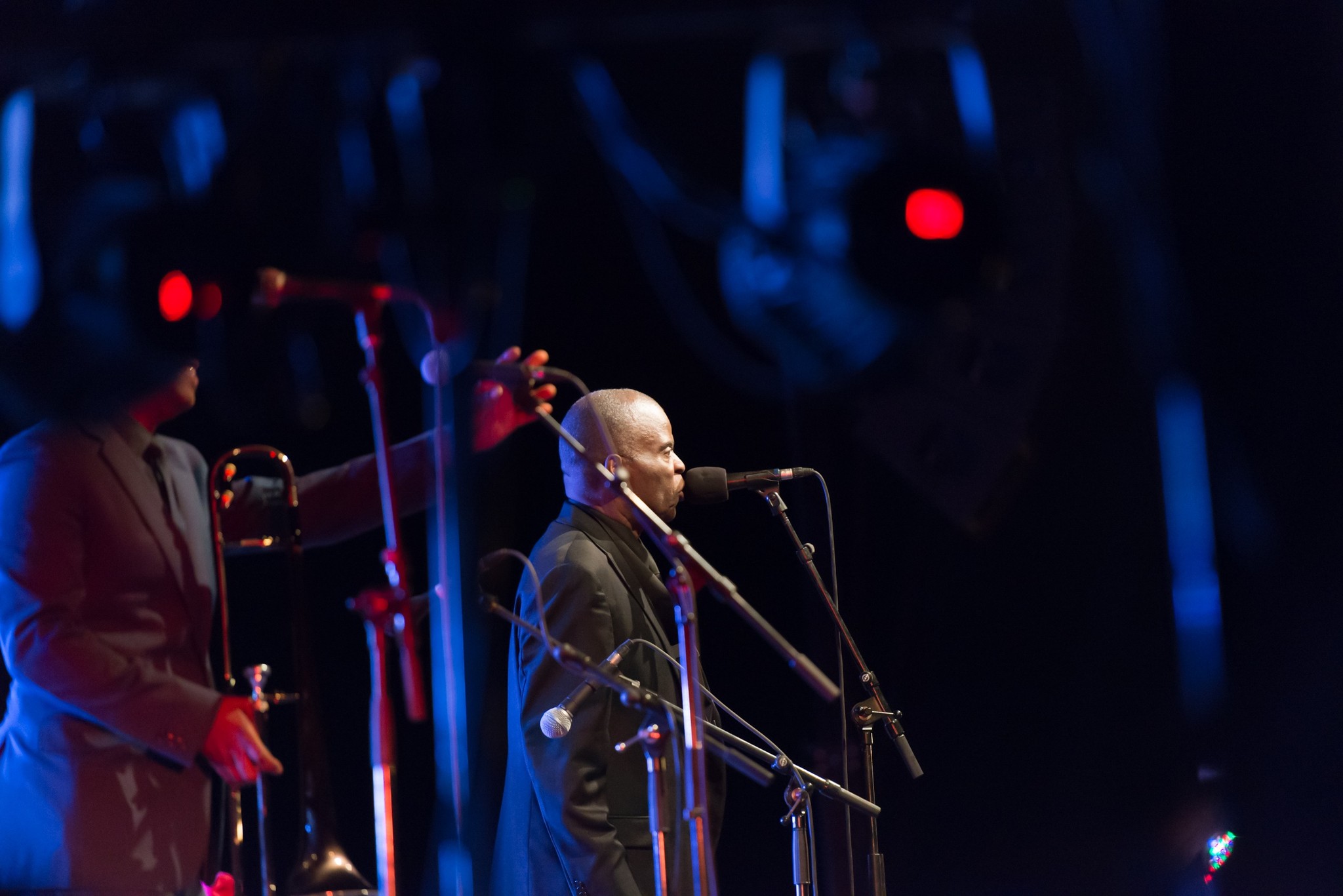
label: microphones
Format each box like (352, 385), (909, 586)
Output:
(421, 351), (571, 384)
(540, 639), (635, 738)
(254, 269), (400, 307)
(384, 550), (507, 636)
(686, 467), (815, 503)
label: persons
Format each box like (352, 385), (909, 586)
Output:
(0, 269), (557, 896)
(487, 386), (726, 896)
